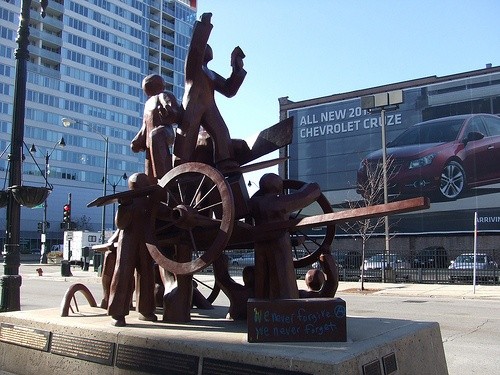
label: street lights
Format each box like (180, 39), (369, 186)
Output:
(101, 172), (128, 230)
(29, 137), (67, 264)
(61, 117), (109, 272)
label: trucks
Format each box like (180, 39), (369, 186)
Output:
(63, 229), (116, 266)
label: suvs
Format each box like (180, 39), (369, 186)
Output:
(231, 252), (255, 266)
(413, 247), (447, 268)
(331, 250), (362, 278)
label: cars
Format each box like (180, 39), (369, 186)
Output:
(360, 253), (411, 279)
(449, 253), (498, 283)
(355, 112), (499, 199)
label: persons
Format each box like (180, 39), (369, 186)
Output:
(248, 173), (322, 298)
(131, 73), (178, 178)
(107, 173), (158, 326)
(173, 12), (247, 175)
